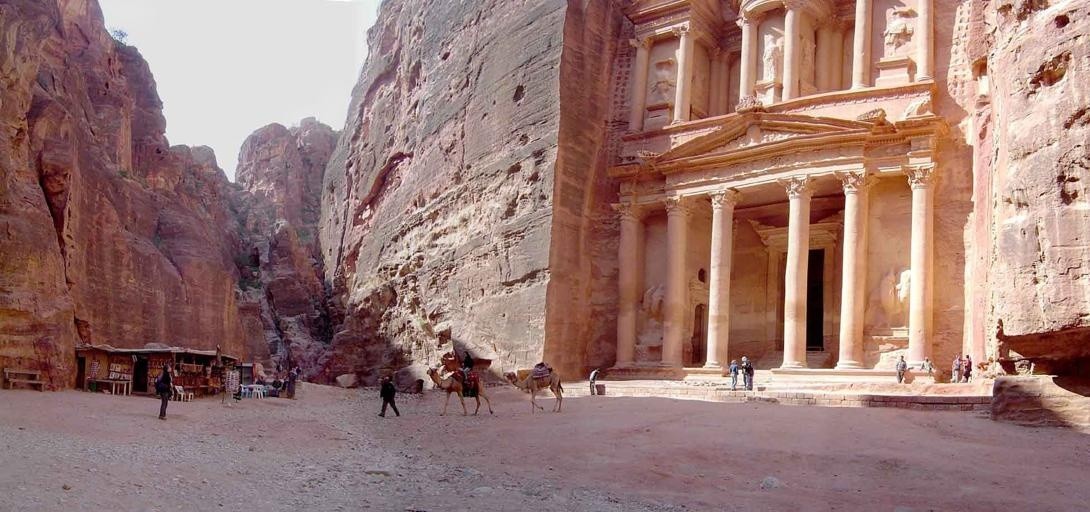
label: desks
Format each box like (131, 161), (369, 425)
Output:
(111, 380), (133, 396)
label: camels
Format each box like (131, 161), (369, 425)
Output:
(503, 369), (564, 413)
(427, 368), (493, 415)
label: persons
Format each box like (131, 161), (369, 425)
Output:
(741, 355), (755, 391)
(919, 356), (934, 371)
(896, 355), (907, 384)
(729, 359), (739, 391)
(159, 364), (174, 421)
(949, 354), (972, 383)
(378, 375), (401, 418)
(458, 350), (474, 384)
(254, 357), (300, 400)
(588, 368), (600, 397)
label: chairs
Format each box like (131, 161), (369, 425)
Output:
(240, 383), (264, 399)
